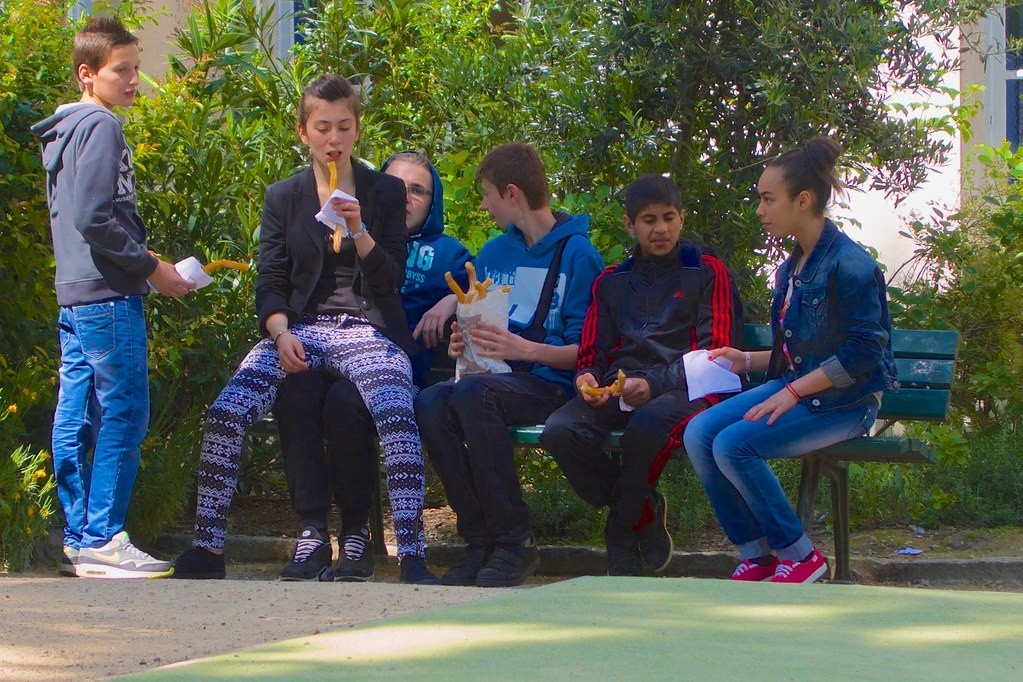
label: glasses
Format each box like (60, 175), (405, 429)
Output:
(406, 185), (433, 203)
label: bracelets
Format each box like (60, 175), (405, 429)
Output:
(348, 222), (368, 240)
(275, 330), (291, 344)
(745, 351), (750, 382)
(786, 383), (800, 399)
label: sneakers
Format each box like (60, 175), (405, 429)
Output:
(170, 545), (226, 579)
(769, 546), (828, 583)
(605, 551), (632, 576)
(638, 490), (673, 573)
(279, 526), (333, 582)
(59, 545), (79, 577)
(475, 533), (542, 586)
(439, 542), (494, 585)
(77, 531), (174, 580)
(399, 555), (439, 585)
(727, 556), (781, 581)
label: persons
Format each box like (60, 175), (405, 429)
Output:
(170, 72), (441, 587)
(538, 173), (741, 576)
(682, 136), (899, 585)
(275, 148), (476, 582)
(414, 141), (605, 590)
(30, 14), (197, 577)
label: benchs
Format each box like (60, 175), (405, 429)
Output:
(240, 330), (957, 583)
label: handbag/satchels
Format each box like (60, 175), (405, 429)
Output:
(423, 314), (547, 380)
(334, 532), (374, 581)
